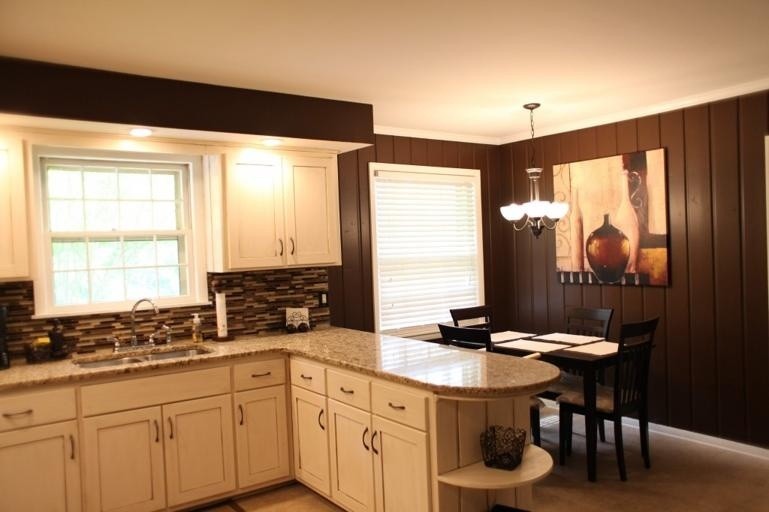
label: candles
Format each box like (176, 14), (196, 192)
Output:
(214, 291), (227, 338)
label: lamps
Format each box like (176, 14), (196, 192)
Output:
(499, 104), (569, 240)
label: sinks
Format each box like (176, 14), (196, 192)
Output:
(143, 343), (214, 362)
(72, 352), (143, 370)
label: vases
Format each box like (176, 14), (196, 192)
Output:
(586, 213), (631, 282)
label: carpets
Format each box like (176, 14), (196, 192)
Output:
(177, 497), (247, 512)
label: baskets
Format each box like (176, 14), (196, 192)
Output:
(480, 423), (526, 471)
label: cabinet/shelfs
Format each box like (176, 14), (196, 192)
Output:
(75, 358), (237, 512)
(287, 357), (331, 502)
(0, 384), (83, 512)
(231, 356), (294, 499)
(326, 364), (435, 512)
(429, 396), (555, 512)
(203, 147), (343, 273)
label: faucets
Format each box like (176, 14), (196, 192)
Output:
(129, 298), (159, 346)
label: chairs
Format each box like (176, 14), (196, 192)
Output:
(557, 313), (660, 484)
(450, 305), (491, 338)
(537, 306), (613, 442)
(438, 324), (544, 448)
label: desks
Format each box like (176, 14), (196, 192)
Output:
(449, 328), (627, 482)
